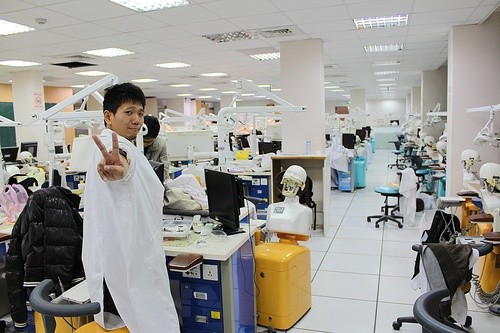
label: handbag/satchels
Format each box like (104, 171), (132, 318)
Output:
(0, 184), (29, 222)
(164, 188), (201, 211)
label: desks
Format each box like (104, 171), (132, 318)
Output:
(0, 200), (257, 333)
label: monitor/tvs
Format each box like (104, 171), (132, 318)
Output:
(1, 147), (19, 162)
(342, 133), (354, 148)
(204, 168), (245, 235)
(357, 129), (365, 141)
(21, 142), (37, 157)
(273, 140), (281, 149)
(49, 166), (60, 187)
(55, 146), (64, 153)
(258, 142), (274, 153)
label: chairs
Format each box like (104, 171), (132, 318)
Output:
(368, 130), (493, 332)
(30, 278), (130, 333)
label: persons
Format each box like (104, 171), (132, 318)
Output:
(138, 115), (169, 182)
(79, 82), (179, 333)
